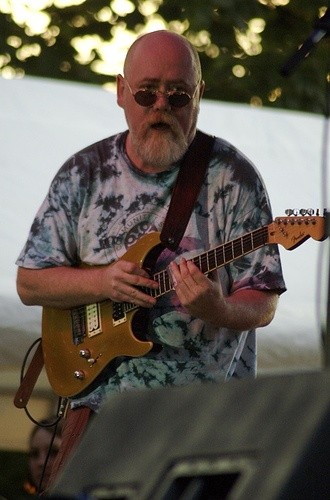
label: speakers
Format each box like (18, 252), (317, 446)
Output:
(47, 368), (330, 500)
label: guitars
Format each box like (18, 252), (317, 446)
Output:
(41, 208), (330, 400)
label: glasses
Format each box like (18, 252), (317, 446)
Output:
(29, 445), (60, 460)
(124, 79), (201, 108)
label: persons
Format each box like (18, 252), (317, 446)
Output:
(15, 30), (287, 492)
(28, 413), (63, 492)
